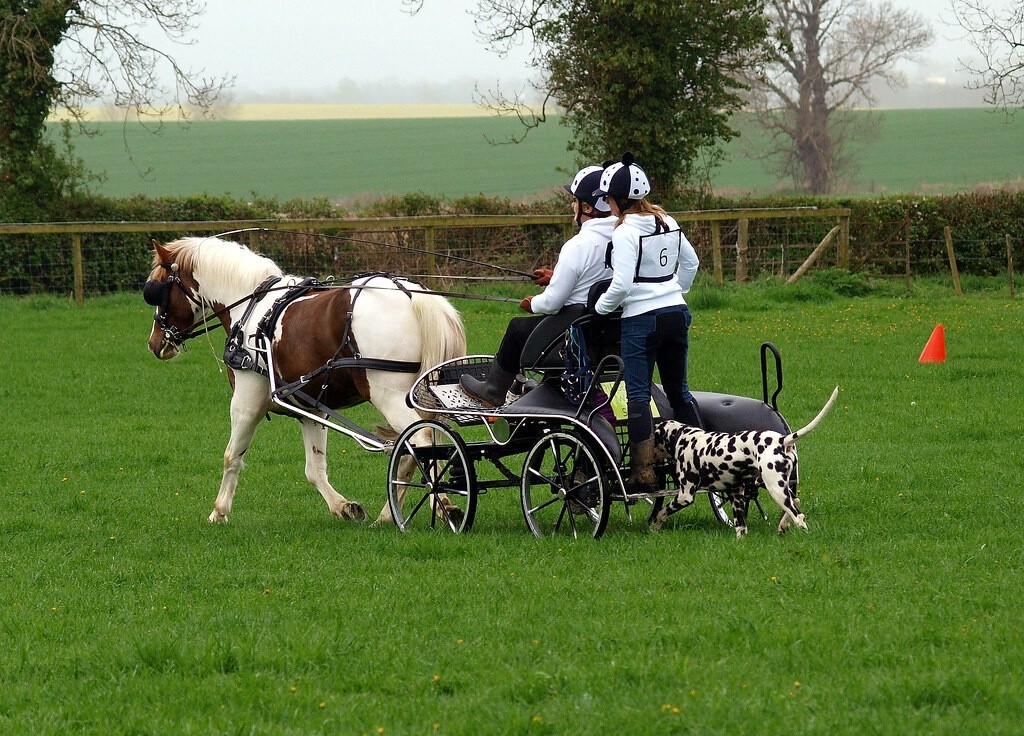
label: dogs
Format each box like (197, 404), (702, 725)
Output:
(649, 385), (839, 540)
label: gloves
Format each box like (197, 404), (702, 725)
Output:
(520, 296), (534, 313)
(533, 269), (554, 285)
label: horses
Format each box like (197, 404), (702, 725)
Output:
(143, 236), (468, 530)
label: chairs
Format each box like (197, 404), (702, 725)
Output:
(520, 277), (625, 382)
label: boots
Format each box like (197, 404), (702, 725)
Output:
(459, 353), (516, 408)
(674, 398), (709, 432)
(612, 401), (661, 493)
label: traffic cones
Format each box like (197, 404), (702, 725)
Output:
(917, 325), (948, 363)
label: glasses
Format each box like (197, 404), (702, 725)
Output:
(602, 196), (614, 204)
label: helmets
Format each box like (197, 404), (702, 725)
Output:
(592, 152), (651, 199)
(563, 160), (615, 212)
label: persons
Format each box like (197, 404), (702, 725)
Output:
(460, 166), (619, 410)
(591, 152), (699, 495)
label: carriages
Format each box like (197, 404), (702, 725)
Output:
(142, 237), (797, 542)
